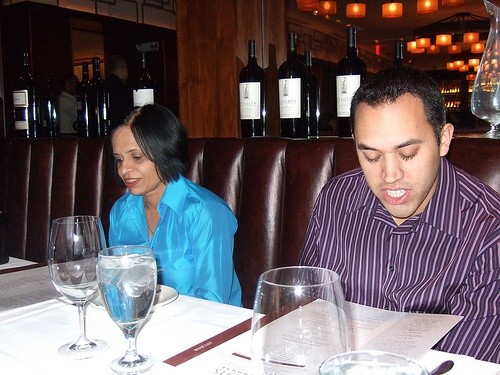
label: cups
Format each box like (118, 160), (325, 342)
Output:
(250, 266), (431, 375)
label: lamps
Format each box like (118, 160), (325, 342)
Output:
(295, 0), (500, 92)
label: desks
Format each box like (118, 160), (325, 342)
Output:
(0, 259), (500, 375)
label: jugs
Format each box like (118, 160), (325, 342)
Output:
(470, 0), (500, 140)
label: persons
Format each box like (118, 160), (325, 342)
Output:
(287, 65), (500, 364)
(108, 103), (243, 308)
(60, 54), (131, 133)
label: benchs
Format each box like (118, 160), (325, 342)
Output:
(0, 133), (500, 314)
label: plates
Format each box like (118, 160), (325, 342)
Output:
(91, 285), (179, 312)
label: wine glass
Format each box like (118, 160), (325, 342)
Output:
(96, 245), (156, 375)
(47, 216), (108, 354)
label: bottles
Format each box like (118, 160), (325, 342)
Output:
(132, 51), (154, 110)
(392, 41), (404, 68)
(304, 49), (322, 135)
(76, 57), (111, 132)
(277, 31), (310, 137)
(13, 50), (57, 137)
(237, 39), (267, 137)
(333, 26), (367, 138)
